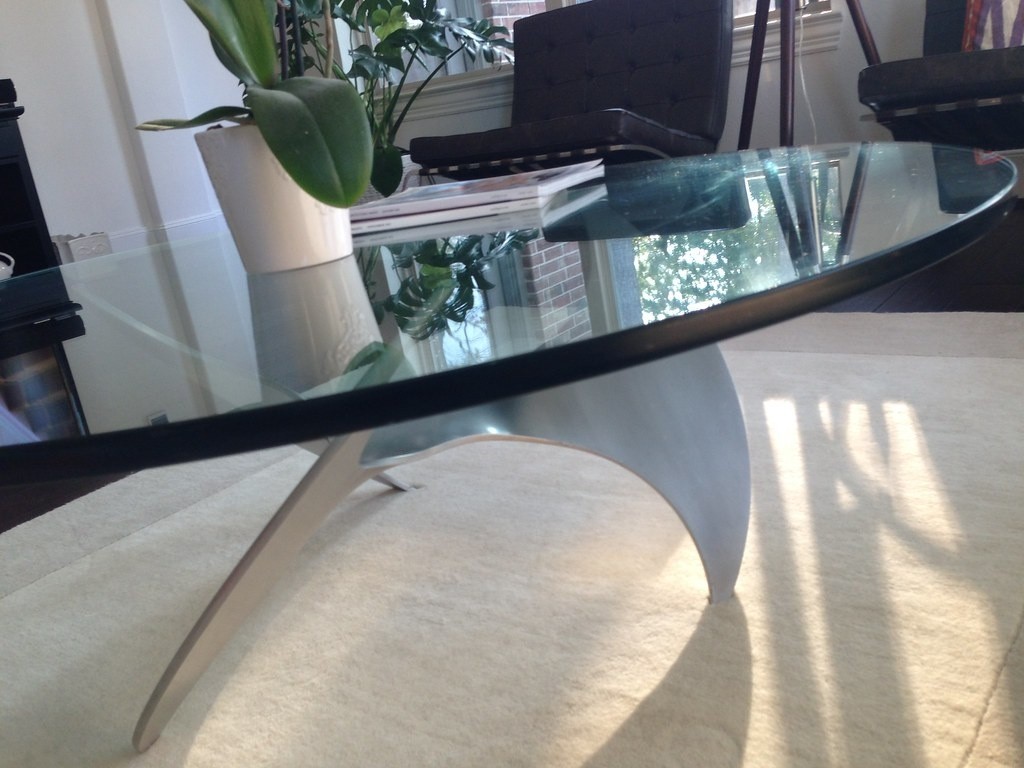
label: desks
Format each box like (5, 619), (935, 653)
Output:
(2, 136), (1020, 482)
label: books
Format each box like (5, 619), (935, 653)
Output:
(354, 185), (608, 247)
(350, 158), (605, 235)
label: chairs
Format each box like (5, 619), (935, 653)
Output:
(857, 4), (1024, 287)
(408, 1), (737, 192)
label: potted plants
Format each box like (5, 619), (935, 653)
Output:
(131, 1), (374, 277)
(241, 252), (429, 458)
